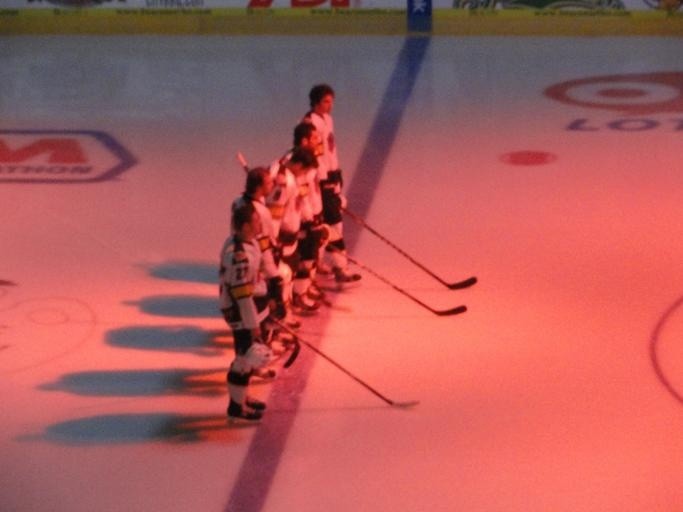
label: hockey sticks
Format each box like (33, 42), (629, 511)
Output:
(324, 242), (467, 316)
(339, 206), (477, 289)
(270, 314), (419, 407)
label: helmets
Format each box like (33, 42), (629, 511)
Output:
(243, 343), (273, 368)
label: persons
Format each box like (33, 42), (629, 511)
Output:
(281, 122), (362, 285)
(218, 201), (271, 420)
(233, 168), (275, 380)
(298, 84), (346, 216)
(267, 146), (326, 310)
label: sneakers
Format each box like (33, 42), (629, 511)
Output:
(252, 369), (276, 378)
(335, 268), (361, 282)
(293, 289), (326, 310)
(227, 396), (265, 419)
(288, 320), (300, 328)
